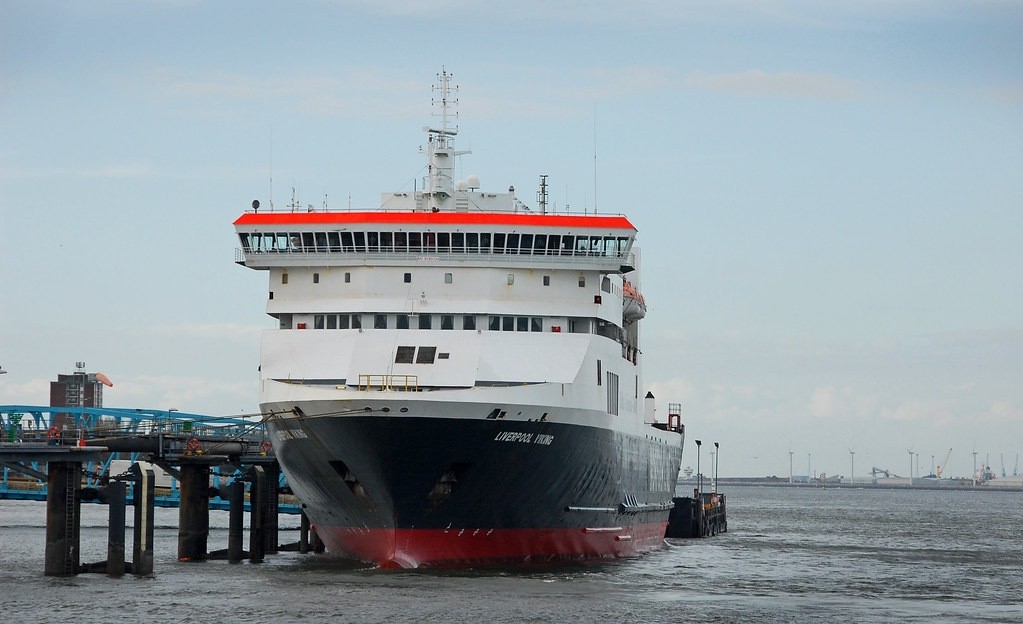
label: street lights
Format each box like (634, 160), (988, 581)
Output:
(909, 451), (914, 486)
(695, 439), (701, 496)
(788, 452), (795, 483)
(850, 451), (856, 485)
(715, 441), (719, 496)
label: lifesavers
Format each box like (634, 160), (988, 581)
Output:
(188, 439), (201, 449)
(47, 426), (60, 438)
(261, 442), (271, 452)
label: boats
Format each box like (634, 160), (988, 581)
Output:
(232, 55), (687, 571)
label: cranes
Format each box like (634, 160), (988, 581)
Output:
(929, 447), (952, 478)
(984, 451), (1020, 477)
